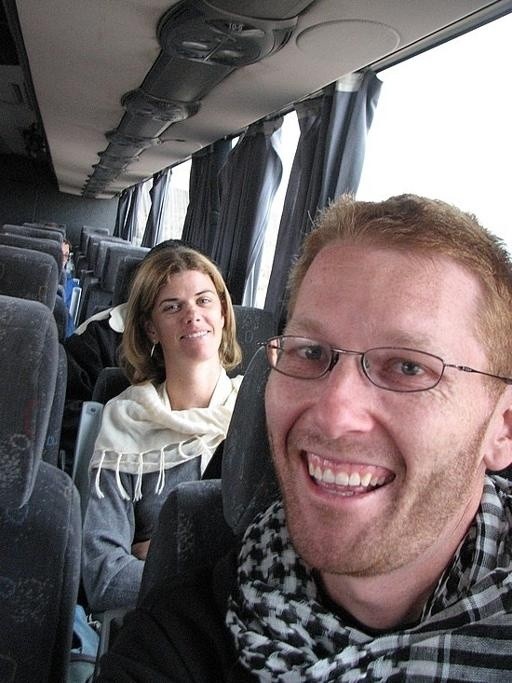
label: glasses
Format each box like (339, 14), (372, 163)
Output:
(256, 332), (511, 394)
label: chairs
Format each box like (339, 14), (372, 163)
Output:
(0, 222), (283, 683)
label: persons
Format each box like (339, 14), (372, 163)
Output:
(58, 237), (81, 340)
(65, 239), (245, 680)
(56, 235), (206, 465)
(89, 190), (512, 683)
